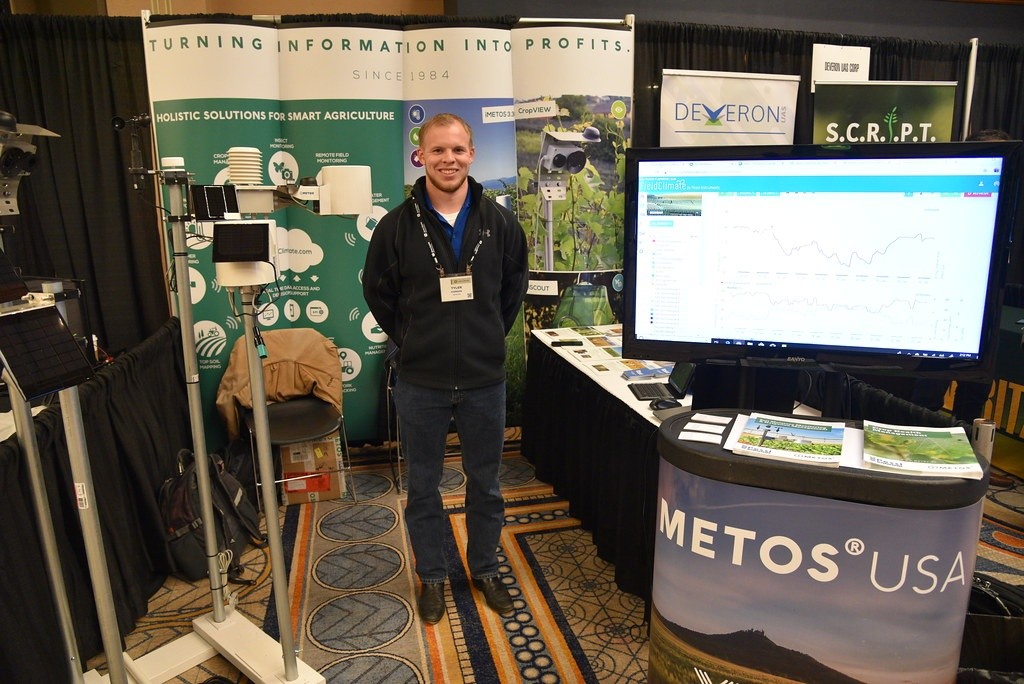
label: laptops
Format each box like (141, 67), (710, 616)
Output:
(631, 362), (696, 400)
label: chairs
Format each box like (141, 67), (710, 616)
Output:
(235, 328), (358, 518)
(384, 338), (402, 495)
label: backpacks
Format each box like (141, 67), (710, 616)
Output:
(159, 448), (267, 585)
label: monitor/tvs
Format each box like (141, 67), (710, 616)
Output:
(0, 305), (98, 402)
(622, 138), (1024, 369)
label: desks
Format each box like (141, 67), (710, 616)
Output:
(647, 408), (991, 684)
(520, 322), (824, 637)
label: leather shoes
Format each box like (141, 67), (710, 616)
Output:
(418, 582), (446, 626)
(470, 575), (516, 615)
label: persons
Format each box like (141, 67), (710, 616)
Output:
(360, 116), (531, 627)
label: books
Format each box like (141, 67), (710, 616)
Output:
(863, 420), (984, 481)
(733, 412), (846, 468)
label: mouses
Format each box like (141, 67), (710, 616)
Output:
(650, 399), (682, 411)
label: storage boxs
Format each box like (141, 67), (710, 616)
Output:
(280, 425), (347, 506)
